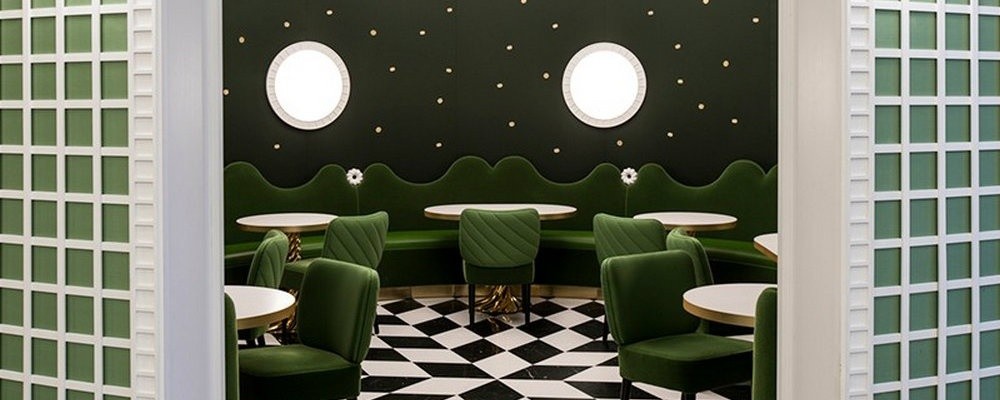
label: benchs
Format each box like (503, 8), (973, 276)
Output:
(225, 155), (778, 303)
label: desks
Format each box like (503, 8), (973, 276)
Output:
(424, 203), (576, 314)
(225, 285), (296, 346)
(683, 282), (778, 337)
(753, 233), (778, 262)
(235, 212), (340, 335)
(634, 212), (738, 236)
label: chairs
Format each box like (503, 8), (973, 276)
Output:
(600, 249), (753, 400)
(459, 209), (541, 330)
(666, 227), (715, 287)
(593, 214), (667, 341)
(286, 211), (390, 336)
(248, 229), (288, 287)
